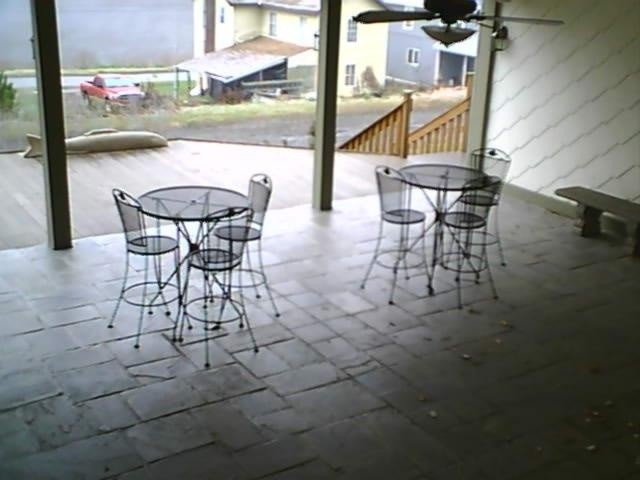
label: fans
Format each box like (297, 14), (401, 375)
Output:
(351, 1), (566, 29)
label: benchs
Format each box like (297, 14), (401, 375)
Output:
(556, 185), (640, 255)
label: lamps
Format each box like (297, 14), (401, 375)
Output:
(420, 21), (476, 48)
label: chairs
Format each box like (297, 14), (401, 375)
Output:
(107, 173), (286, 369)
(359, 145), (511, 309)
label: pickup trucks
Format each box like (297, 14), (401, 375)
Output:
(79, 72), (146, 114)
